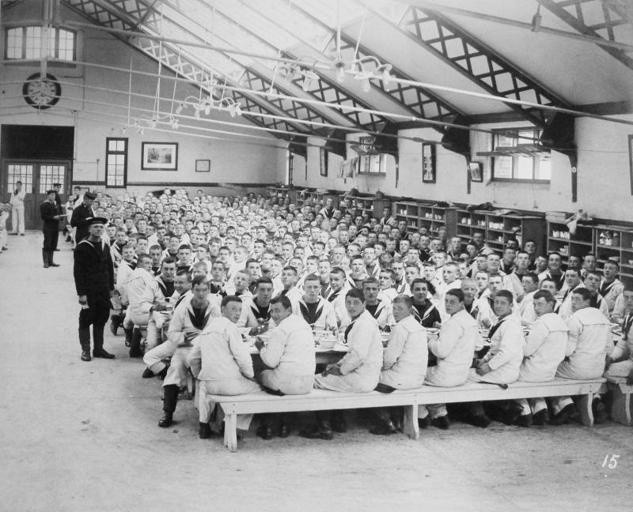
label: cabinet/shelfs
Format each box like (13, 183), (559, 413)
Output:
(336, 194), (390, 219)
(294, 190), (339, 213)
(264, 186), (291, 204)
(453, 206), (546, 261)
(543, 214), (633, 293)
(390, 199), (455, 243)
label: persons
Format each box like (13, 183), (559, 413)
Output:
(63, 186), (632, 440)
(38, 188), (65, 269)
(50, 182), (63, 251)
(8, 181), (26, 234)
(0, 202), (10, 254)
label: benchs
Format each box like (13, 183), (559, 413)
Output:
(126, 323), (163, 359)
(609, 381), (631, 430)
(205, 374), (609, 454)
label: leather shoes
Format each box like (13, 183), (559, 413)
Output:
(81, 350), (115, 361)
(110, 314), (167, 379)
(8, 233), (25, 235)
(0, 247), (8, 253)
(44, 263), (59, 268)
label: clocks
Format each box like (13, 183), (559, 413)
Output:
(23, 73), (60, 108)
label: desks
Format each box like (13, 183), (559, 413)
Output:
(183, 329), (493, 397)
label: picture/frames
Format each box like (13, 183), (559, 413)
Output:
(193, 158), (211, 173)
(468, 160), (484, 184)
(318, 146), (329, 177)
(138, 141), (177, 171)
(421, 141), (436, 185)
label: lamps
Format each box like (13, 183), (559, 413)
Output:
(106, 0), (395, 136)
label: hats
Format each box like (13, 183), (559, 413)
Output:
(53, 183), (61, 186)
(85, 217), (108, 224)
(45, 191), (58, 193)
(85, 192), (97, 200)
